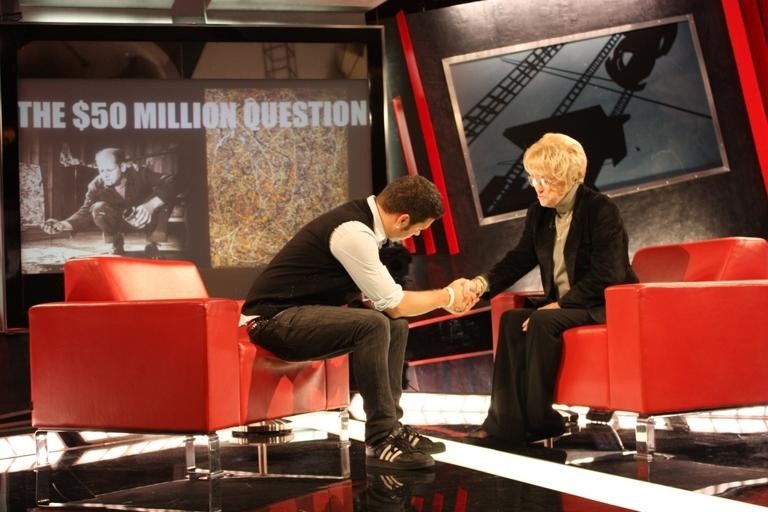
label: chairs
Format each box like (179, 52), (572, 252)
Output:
(490, 237), (766, 465)
(28, 256), (351, 512)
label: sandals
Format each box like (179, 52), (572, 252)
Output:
(464, 411), (566, 442)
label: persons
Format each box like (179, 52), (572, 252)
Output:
(39, 147), (179, 261)
(462, 130), (640, 446)
(237, 173), (476, 471)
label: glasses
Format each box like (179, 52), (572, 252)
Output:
(528, 177), (550, 186)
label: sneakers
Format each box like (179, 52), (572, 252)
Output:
(366, 470), (435, 491)
(365, 425), (446, 469)
(112, 243), (123, 255)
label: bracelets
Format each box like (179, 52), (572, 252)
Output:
(442, 286), (455, 307)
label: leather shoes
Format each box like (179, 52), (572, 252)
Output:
(145, 243), (161, 260)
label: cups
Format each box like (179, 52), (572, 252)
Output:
(123, 207), (146, 230)
